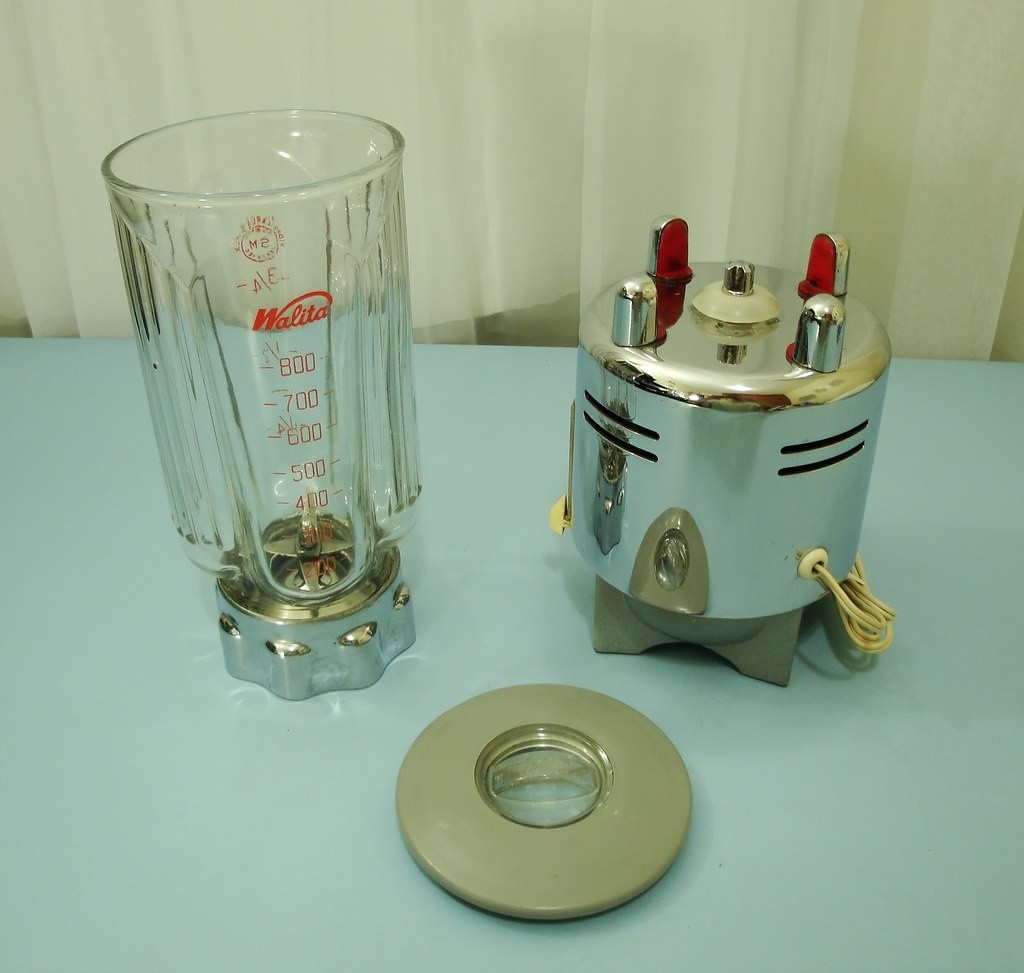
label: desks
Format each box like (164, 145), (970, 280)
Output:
(0, 337), (1024, 973)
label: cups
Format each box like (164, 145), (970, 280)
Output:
(101, 107), (422, 701)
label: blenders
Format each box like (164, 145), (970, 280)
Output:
(549, 216), (892, 688)
